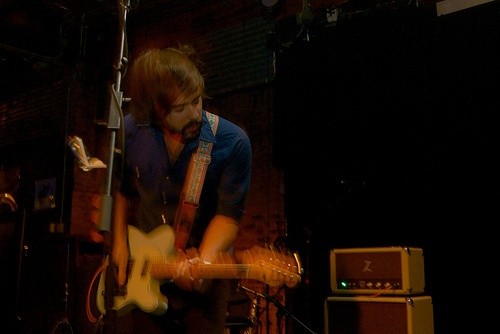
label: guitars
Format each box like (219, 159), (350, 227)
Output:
(95, 223), (302, 318)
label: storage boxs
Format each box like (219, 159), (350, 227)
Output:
(324, 296), (435, 334)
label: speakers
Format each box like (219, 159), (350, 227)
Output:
(330, 247), (425, 296)
(324, 296), (434, 334)
